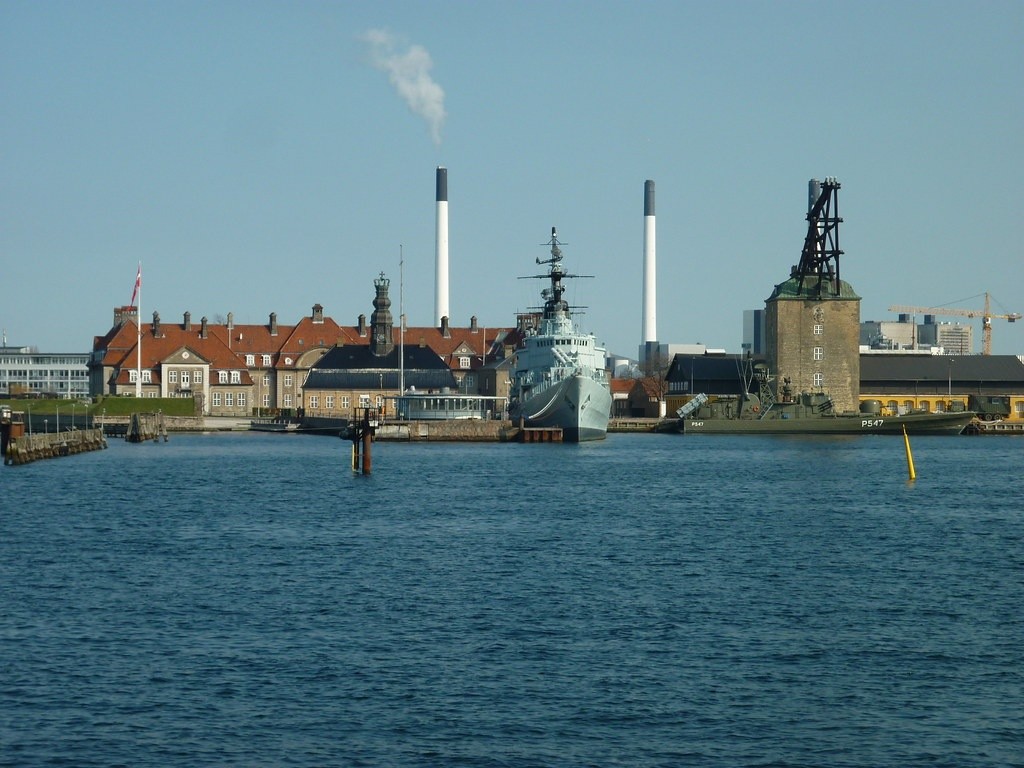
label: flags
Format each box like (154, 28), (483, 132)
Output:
(128, 264), (142, 310)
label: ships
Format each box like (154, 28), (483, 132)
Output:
(672, 347), (977, 436)
(508, 225), (614, 444)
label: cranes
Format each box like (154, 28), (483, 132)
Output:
(890, 291), (1021, 356)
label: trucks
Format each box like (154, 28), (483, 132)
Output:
(948, 392), (1011, 422)
